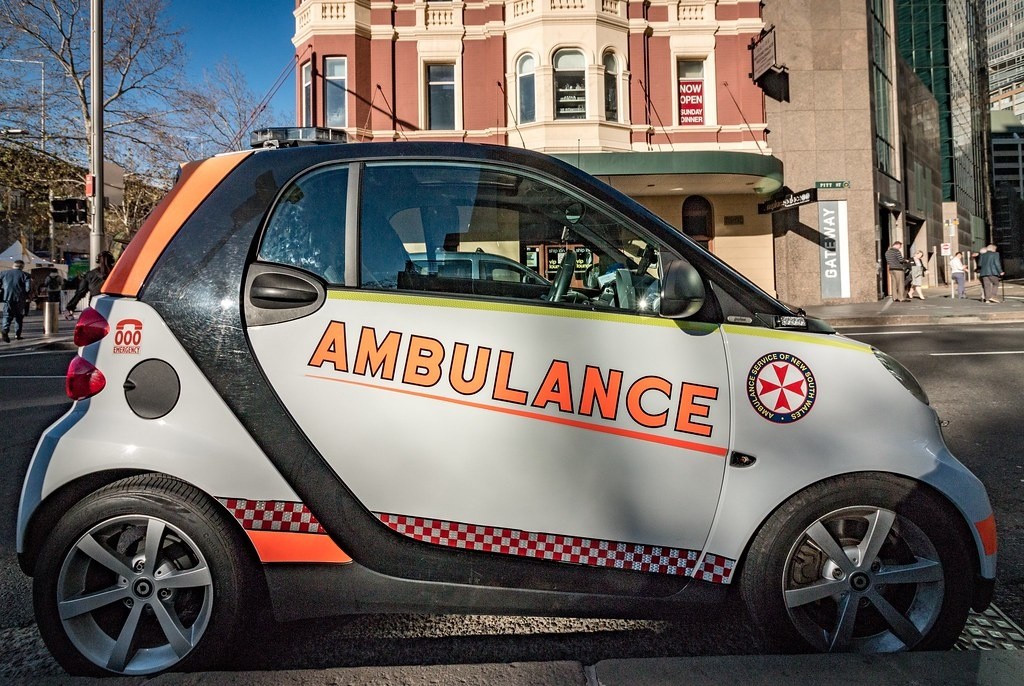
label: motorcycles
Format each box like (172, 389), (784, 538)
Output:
(15, 143), (995, 673)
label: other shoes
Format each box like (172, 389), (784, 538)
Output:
(893, 299), (911, 302)
(2, 334), (10, 343)
(15, 335), (24, 339)
(989, 296), (1000, 302)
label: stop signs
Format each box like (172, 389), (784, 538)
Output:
(942, 244), (950, 250)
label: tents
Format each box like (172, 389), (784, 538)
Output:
(0, 240), (68, 280)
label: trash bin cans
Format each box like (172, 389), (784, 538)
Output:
(44, 301), (59, 333)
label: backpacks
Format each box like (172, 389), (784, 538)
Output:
(48, 275), (58, 290)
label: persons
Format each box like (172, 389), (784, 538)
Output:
(0, 259), (32, 343)
(979, 244), (1004, 304)
(66, 251), (115, 316)
(907, 250), (927, 300)
(885, 241), (912, 302)
(950, 252), (968, 299)
(973, 247), (988, 303)
(45, 268), (64, 315)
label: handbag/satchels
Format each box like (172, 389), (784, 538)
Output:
(905, 274), (912, 286)
